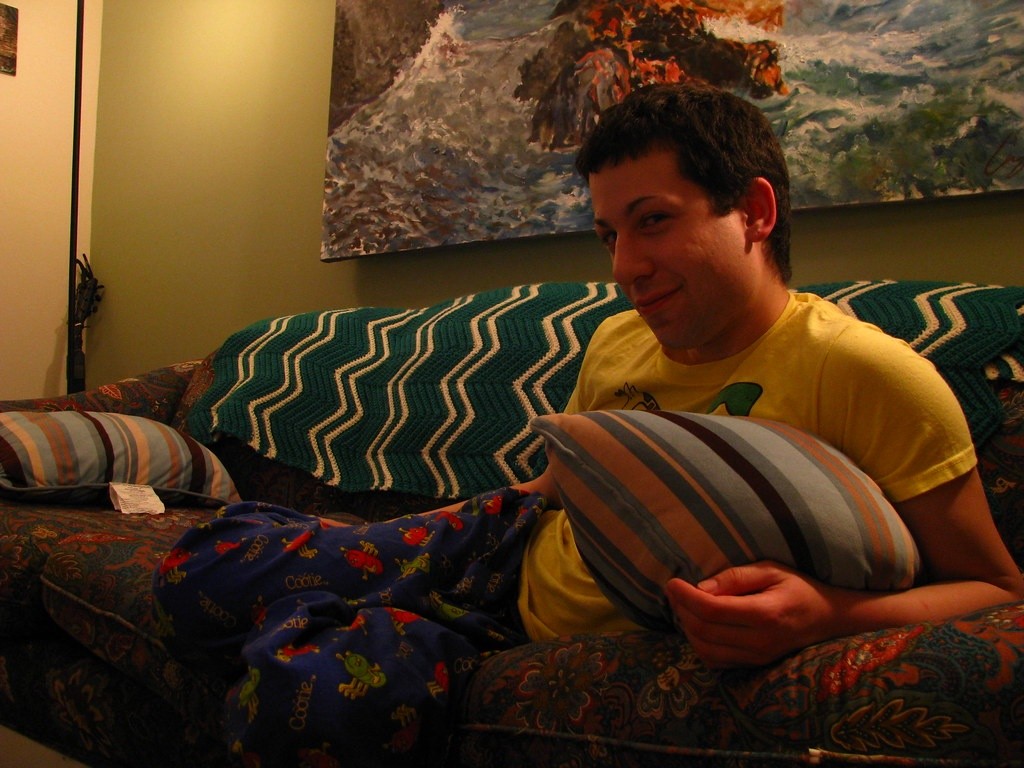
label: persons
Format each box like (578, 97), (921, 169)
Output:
(150, 81), (1024, 668)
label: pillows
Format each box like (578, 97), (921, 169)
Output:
(528, 410), (928, 633)
(0, 410), (244, 513)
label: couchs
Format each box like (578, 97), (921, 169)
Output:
(0, 279), (1024, 768)
